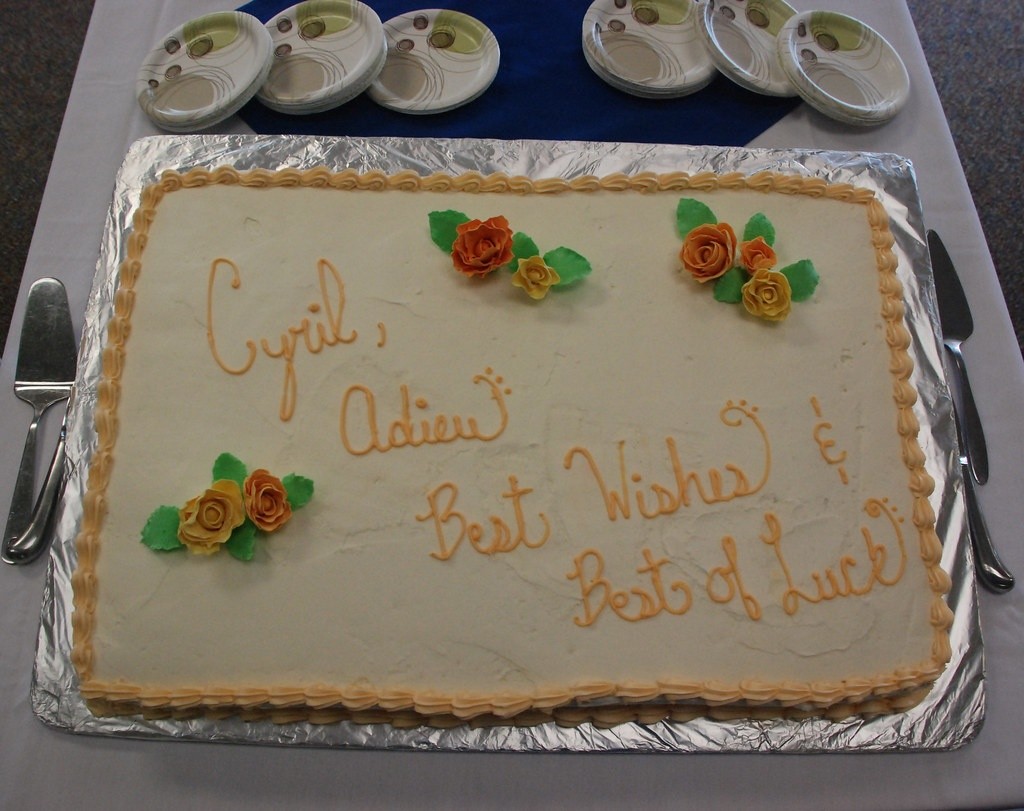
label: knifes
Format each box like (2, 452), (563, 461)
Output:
(926, 228), (988, 485)
(1, 278), (78, 564)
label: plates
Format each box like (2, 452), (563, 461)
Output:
(257, 0), (388, 115)
(582, 0), (713, 98)
(366, 9), (500, 115)
(136, 0), (273, 133)
(775, 10), (910, 128)
(698, 0), (797, 99)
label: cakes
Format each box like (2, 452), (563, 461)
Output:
(69, 165), (953, 731)
